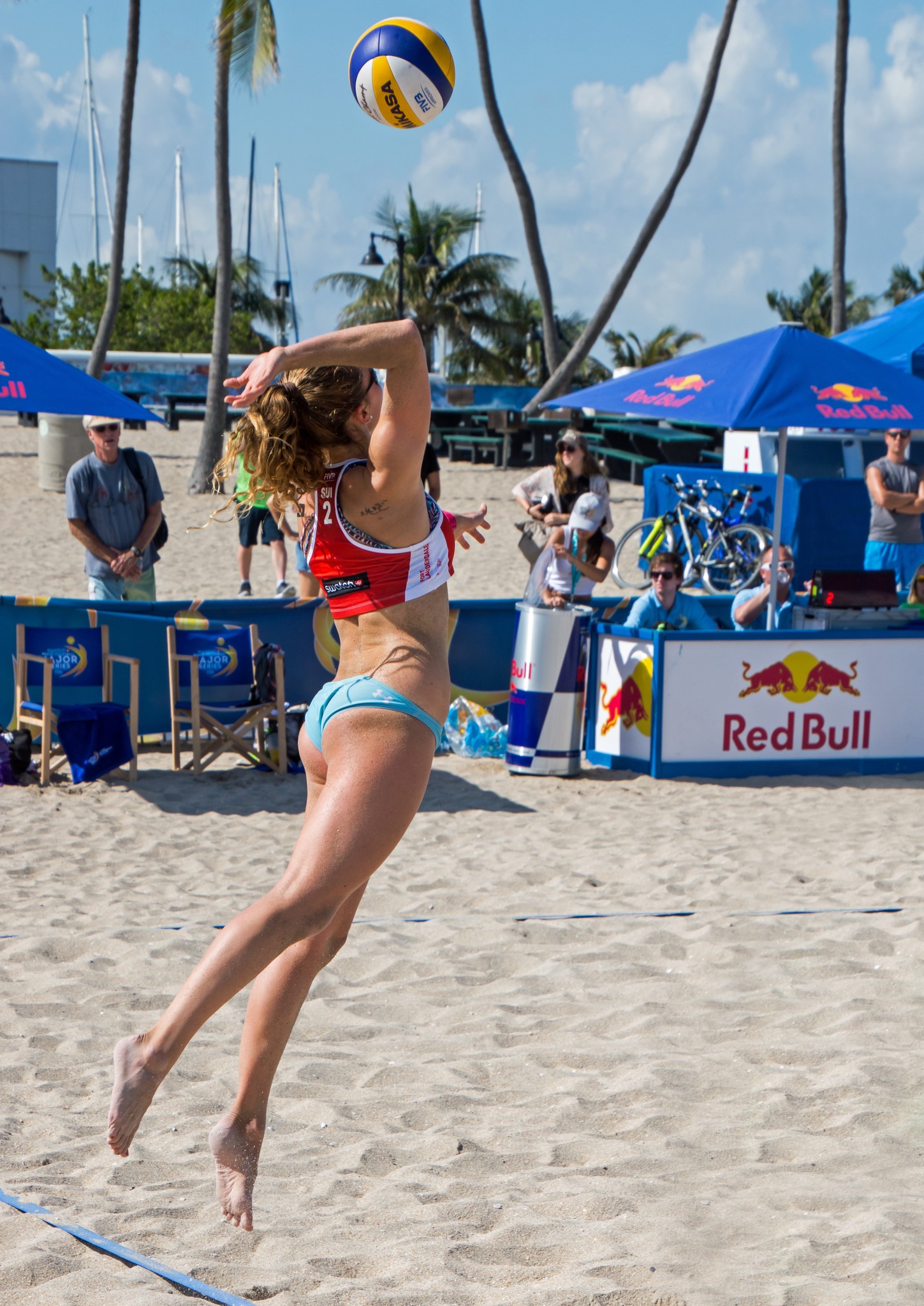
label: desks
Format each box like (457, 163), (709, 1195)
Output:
(665, 417), (721, 429)
(585, 412), (665, 426)
(161, 391), (239, 430)
(469, 414), (571, 465)
(593, 422), (713, 468)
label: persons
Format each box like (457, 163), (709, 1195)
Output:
(422, 439), (442, 506)
(232, 430), (297, 597)
(535, 491), (616, 608)
(512, 429), (614, 535)
(898, 564), (924, 619)
(863, 427), (924, 602)
(105, 320), (491, 1218)
(624, 552), (719, 631)
(66, 415), (164, 605)
(729, 542), (813, 632)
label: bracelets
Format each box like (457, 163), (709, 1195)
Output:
(526, 506), (533, 515)
(131, 544), (142, 557)
(570, 557), (577, 564)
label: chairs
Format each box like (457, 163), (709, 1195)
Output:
(13, 623), (139, 787)
(166, 623), (287, 778)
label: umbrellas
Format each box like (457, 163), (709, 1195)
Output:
(538, 322), (924, 635)
(829, 290), (924, 379)
(0, 326), (165, 422)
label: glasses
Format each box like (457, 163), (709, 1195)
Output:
(96, 423), (118, 433)
(762, 561), (794, 572)
(916, 575), (924, 583)
(558, 444), (576, 454)
(886, 430), (909, 438)
(649, 571), (675, 580)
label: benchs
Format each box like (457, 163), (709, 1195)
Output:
(428, 424), (721, 484)
(156, 405), (249, 413)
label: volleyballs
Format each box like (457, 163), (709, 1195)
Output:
(348, 16), (455, 129)
(265, 730), (279, 763)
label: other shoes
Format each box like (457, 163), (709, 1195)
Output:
(275, 581), (296, 599)
(238, 582), (251, 596)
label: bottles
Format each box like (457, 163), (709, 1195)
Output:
(778, 571), (791, 584)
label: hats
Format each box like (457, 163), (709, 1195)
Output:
(82, 415), (123, 430)
(568, 492), (606, 531)
(556, 428), (583, 449)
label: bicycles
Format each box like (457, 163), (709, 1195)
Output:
(610, 472), (772, 596)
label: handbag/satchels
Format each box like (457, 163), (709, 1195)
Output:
(143, 510), (168, 551)
(444, 695), (508, 760)
(0, 723), (32, 785)
(517, 520), (555, 564)
